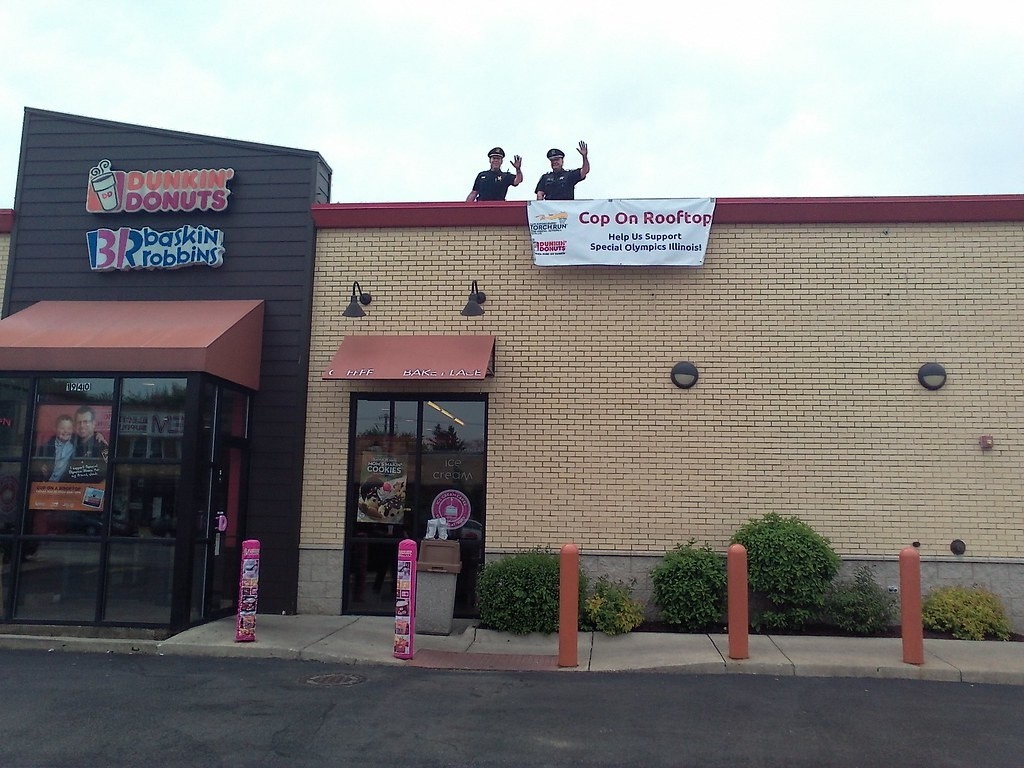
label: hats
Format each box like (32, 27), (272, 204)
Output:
(547, 149), (565, 160)
(488, 147), (505, 158)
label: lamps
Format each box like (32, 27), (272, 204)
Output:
(671, 362), (699, 389)
(342, 281), (371, 318)
(918, 360), (946, 391)
(462, 280), (486, 317)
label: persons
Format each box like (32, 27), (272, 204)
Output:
(466, 148), (523, 203)
(535, 140), (590, 200)
(34, 407), (110, 483)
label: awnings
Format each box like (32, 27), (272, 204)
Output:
(322, 335), (496, 381)
(0, 301), (264, 390)
